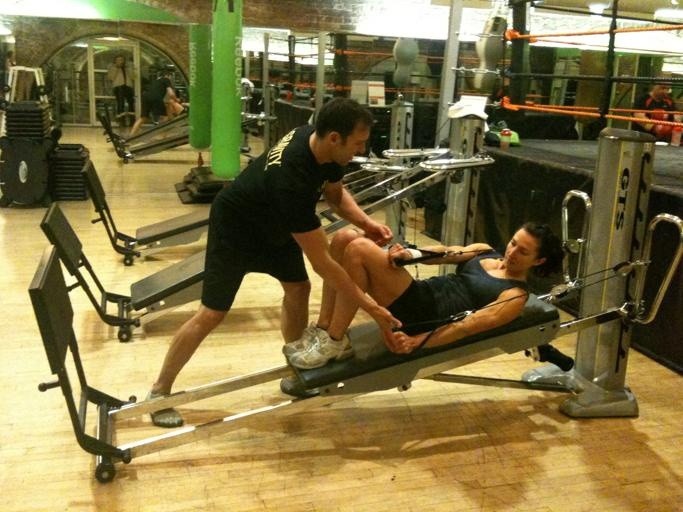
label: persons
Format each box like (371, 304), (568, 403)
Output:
(630, 77), (679, 139)
(108, 55), (135, 124)
(282, 223), (565, 370)
(128, 70), (181, 141)
(144, 96), (394, 428)
(166, 96), (190, 117)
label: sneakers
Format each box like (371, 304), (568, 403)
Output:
(289, 330), (353, 369)
(282, 327), (326, 357)
